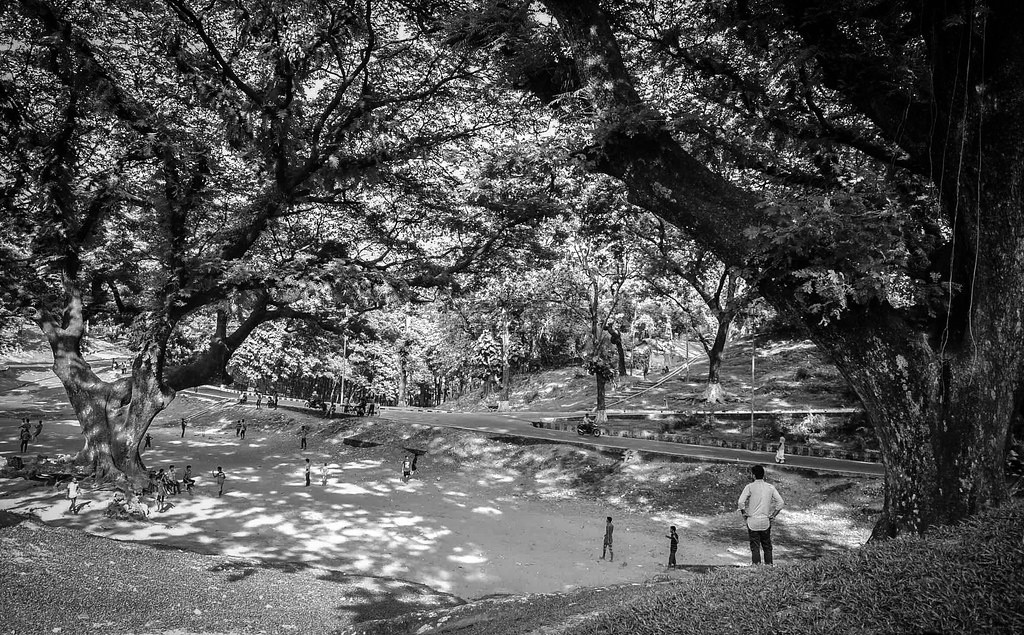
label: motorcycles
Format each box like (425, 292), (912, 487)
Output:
(577, 423), (600, 437)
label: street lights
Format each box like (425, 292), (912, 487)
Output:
(750, 305), (757, 440)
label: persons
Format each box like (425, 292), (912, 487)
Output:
(309, 390), (375, 418)
(600, 517), (615, 562)
(305, 458), (311, 486)
(320, 462), (329, 486)
(143, 433), (153, 450)
(16, 417), (43, 453)
(112, 357), (133, 375)
(238, 391), (278, 410)
(582, 413), (594, 432)
(148, 464), (226, 512)
(737, 465), (784, 565)
(661, 366), (669, 380)
(401, 453), (420, 483)
(181, 418), (187, 438)
(644, 364), (648, 377)
(775, 432), (786, 463)
(234, 418), (246, 440)
(66, 477), (80, 515)
(665, 526), (679, 572)
(296, 426), (309, 449)
